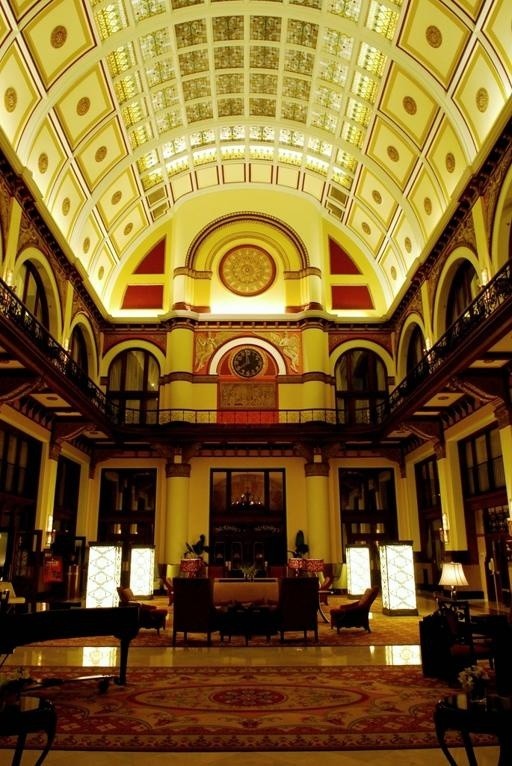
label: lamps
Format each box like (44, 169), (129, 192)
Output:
(83, 540), (156, 609)
(179, 558), (202, 578)
(288, 557), (324, 578)
(344, 540), (470, 617)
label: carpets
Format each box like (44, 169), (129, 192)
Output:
(0, 662), (498, 755)
(23, 592), (512, 649)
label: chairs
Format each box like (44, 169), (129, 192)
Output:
(116, 576), (221, 646)
(277, 574), (380, 644)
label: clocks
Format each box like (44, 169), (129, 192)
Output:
(232, 348), (263, 378)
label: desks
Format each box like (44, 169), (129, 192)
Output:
(0, 695), (58, 766)
(432, 697), (510, 765)
(222, 609), (278, 646)
